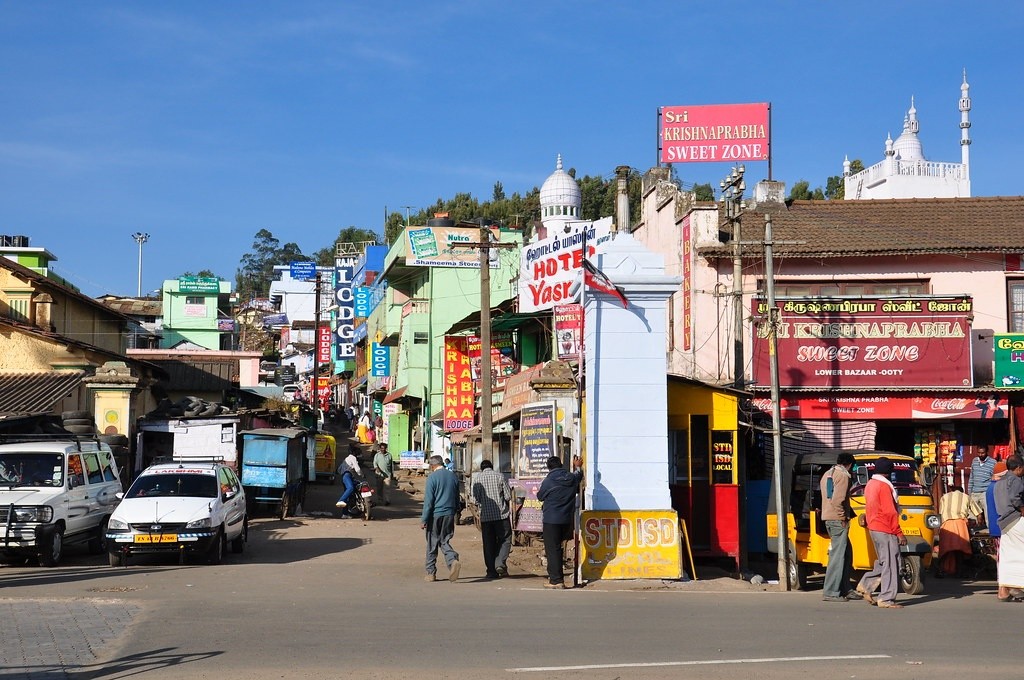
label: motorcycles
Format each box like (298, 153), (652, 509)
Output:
(767, 451), (943, 595)
(313, 434), (337, 484)
(351, 477), (376, 521)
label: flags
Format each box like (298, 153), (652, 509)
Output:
(583, 258), (627, 309)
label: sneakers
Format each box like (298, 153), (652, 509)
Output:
(847, 590), (863, 600)
(822, 596), (847, 601)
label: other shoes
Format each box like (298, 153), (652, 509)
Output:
(935, 571), (955, 578)
(448, 559), (462, 583)
(336, 501), (347, 508)
(484, 575), (499, 579)
(544, 582), (565, 589)
(341, 514), (352, 519)
(856, 590), (876, 605)
(424, 573), (436, 582)
(496, 566), (508, 579)
(885, 604), (903, 608)
(386, 501), (391, 505)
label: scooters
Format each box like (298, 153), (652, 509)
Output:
(346, 438), (365, 466)
(329, 410), (336, 425)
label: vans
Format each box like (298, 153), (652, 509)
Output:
(306, 407), (325, 434)
(260, 361), (279, 380)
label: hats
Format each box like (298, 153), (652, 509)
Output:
(873, 457), (895, 474)
(993, 462), (1009, 477)
(547, 456), (562, 469)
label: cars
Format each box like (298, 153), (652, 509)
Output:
(105, 455), (249, 565)
(282, 384), (302, 398)
(0, 433), (124, 567)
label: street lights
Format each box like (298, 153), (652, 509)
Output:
(132, 230), (151, 298)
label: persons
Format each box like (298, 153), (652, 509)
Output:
(856, 456), (907, 609)
(336, 448), (366, 518)
(345, 403), (371, 444)
(373, 443), (394, 505)
(472, 460), (512, 578)
(938, 442), (1024, 601)
(414, 421), (426, 451)
(420, 455), (461, 582)
(519, 446), (529, 471)
(536, 456), (583, 589)
(820, 453), (863, 602)
(444, 458), (454, 471)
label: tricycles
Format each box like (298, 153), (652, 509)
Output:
(238, 427), (308, 519)
